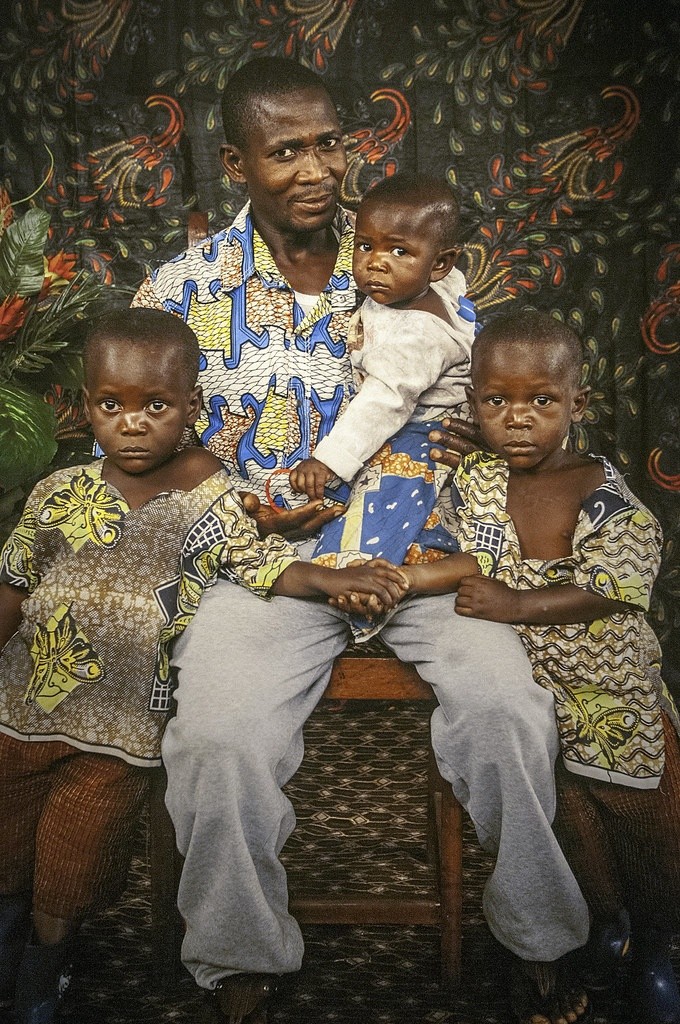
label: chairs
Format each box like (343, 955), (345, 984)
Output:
(148, 214), (464, 999)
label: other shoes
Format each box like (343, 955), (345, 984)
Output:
(495, 947), (593, 1024)
(216, 976), (272, 1024)
(582, 911), (678, 990)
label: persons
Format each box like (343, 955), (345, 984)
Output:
(89, 56), (592, 1022)
(1, 309), (403, 1023)
(290, 164), (476, 570)
(329, 310), (679, 1023)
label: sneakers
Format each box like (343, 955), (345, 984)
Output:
(1, 927), (76, 1024)
